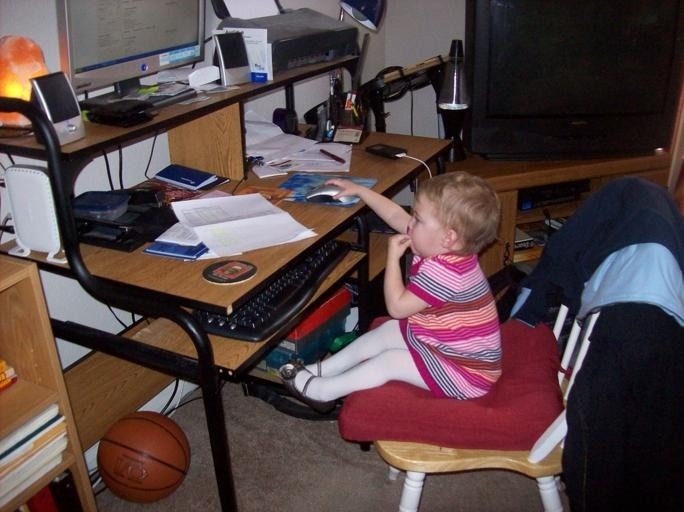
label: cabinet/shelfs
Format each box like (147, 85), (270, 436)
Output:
(472, 170), (673, 294)
(0, 253), (98, 510)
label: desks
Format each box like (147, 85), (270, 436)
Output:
(0, 53), (454, 511)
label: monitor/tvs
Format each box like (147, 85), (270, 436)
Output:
(55, 0), (206, 111)
(458, 0), (684, 160)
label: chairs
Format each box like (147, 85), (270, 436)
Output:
(376, 175), (679, 509)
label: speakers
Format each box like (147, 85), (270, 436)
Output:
(215, 32), (252, 86)
(29, 71), (85, 147)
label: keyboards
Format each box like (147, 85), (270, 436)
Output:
(189, 240), (351, 341)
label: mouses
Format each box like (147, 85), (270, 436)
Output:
(306, 185), (352, 204)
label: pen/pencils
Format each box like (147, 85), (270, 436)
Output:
(320, 149), (346, 164)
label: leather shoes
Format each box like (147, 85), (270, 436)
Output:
(280, 361), (337, 414)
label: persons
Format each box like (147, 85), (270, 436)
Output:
(277, 168), (506, 413)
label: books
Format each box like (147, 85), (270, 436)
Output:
(0, 401), (68, 506)
(242, 110), (361, 178)
(137, 164), (314, 263)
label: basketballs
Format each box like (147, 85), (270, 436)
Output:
(98, 411), (191, 503)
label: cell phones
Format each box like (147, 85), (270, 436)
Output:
(365, 143), (408, 159)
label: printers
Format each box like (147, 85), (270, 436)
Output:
(211, 0), (359, 74)
